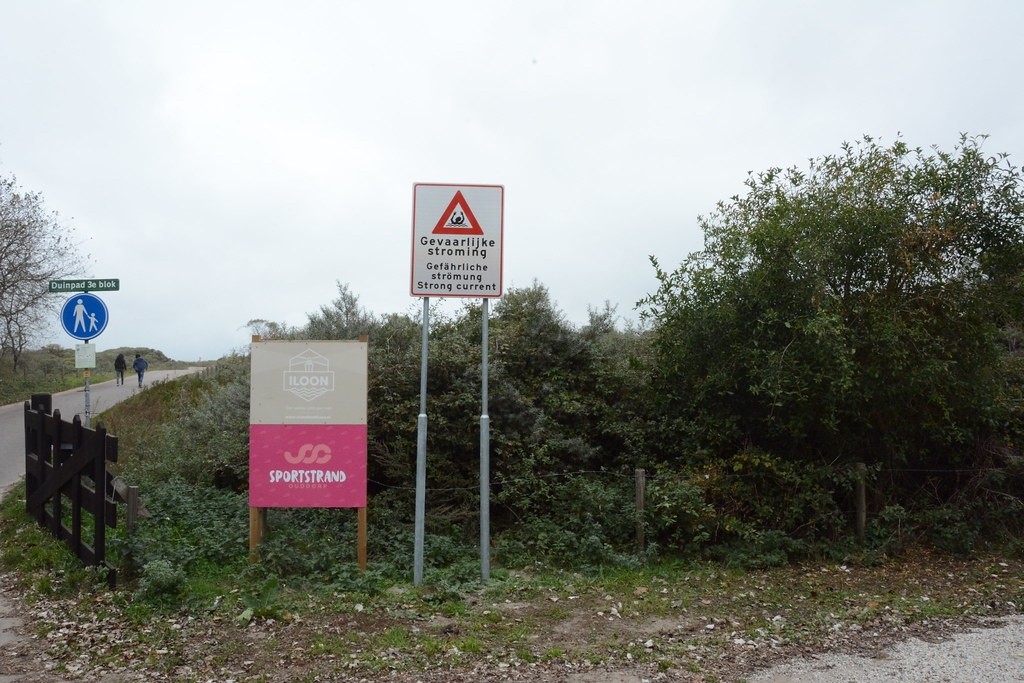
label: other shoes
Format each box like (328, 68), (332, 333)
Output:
(139, 383), (143, 388)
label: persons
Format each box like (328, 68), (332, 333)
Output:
(133, 353), (148, 388)
(114, 353), (128, 387)
